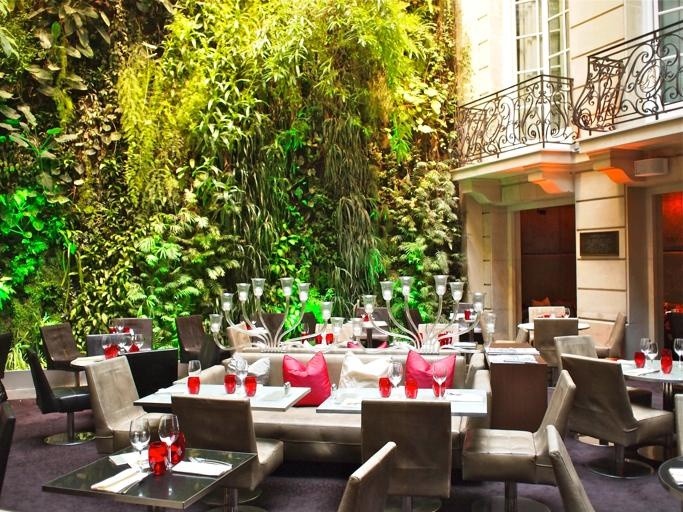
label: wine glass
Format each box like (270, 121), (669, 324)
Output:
(101, 318), (145, 353)
(158, 414), (180, 472)
(640, 337), (683, 369)
(235, 360), (249, 395)
(387, 362), (404, 400)
(187, 359), (202, 378)
(432, 364), (448, 402)
(128, 418), (151, 467)
(562, 308), (571, 318)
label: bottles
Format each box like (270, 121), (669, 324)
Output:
(283, 380), (292, 397)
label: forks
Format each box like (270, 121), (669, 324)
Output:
(187, 455), (229, 467)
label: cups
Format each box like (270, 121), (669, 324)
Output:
(148, 441), (168, 477)
(379, 377), (391, 398)
(162, 432), (186, 466)
(464, 310), (471, 320)
(364, 314), (369, 322)
(634, 348), (673, 374)
(405, 376), (419, 399)
(187, 374), (258, 397)
(432, 379), (446, 398)
